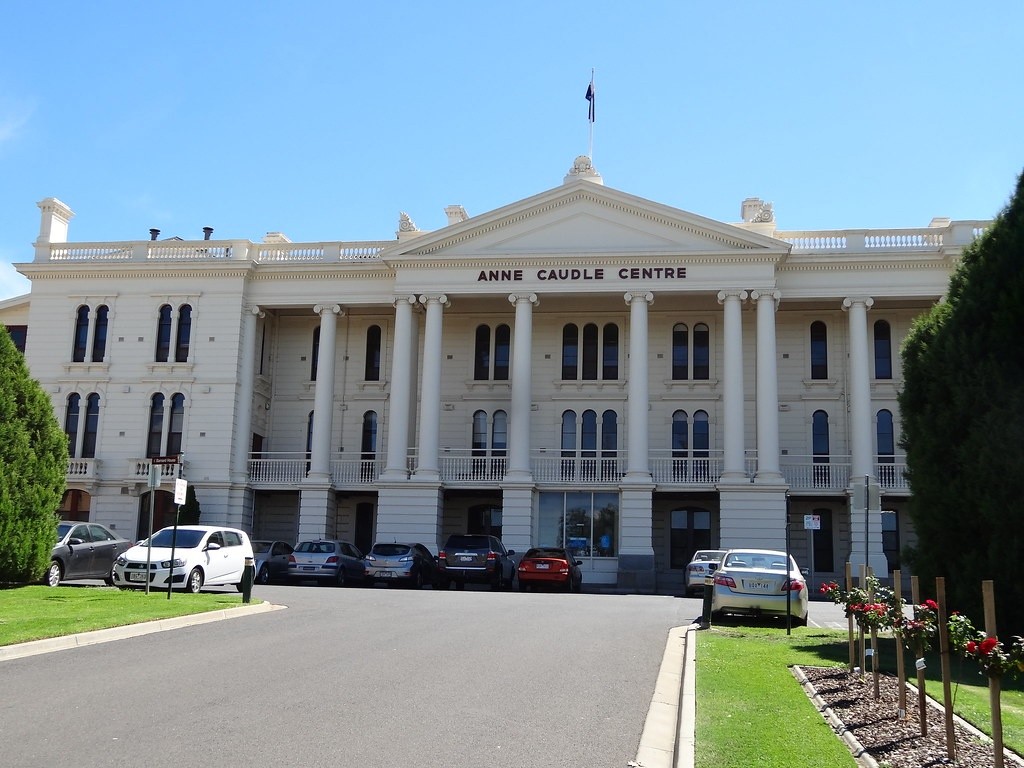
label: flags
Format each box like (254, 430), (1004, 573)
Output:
(585, 81), (595, 122)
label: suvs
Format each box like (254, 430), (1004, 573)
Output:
(437, 535), (516, 592)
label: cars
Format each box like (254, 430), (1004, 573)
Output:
(517, 547), (583, 593)
(112, 525), (257, 596)
(249, 540), (296, 586)
(364, 541), (440, 590)
(287, 539), (367, 588)
(683, 550), (739, 598)
(713, 549), (810, 628)
(38, 521), (133, 586)
